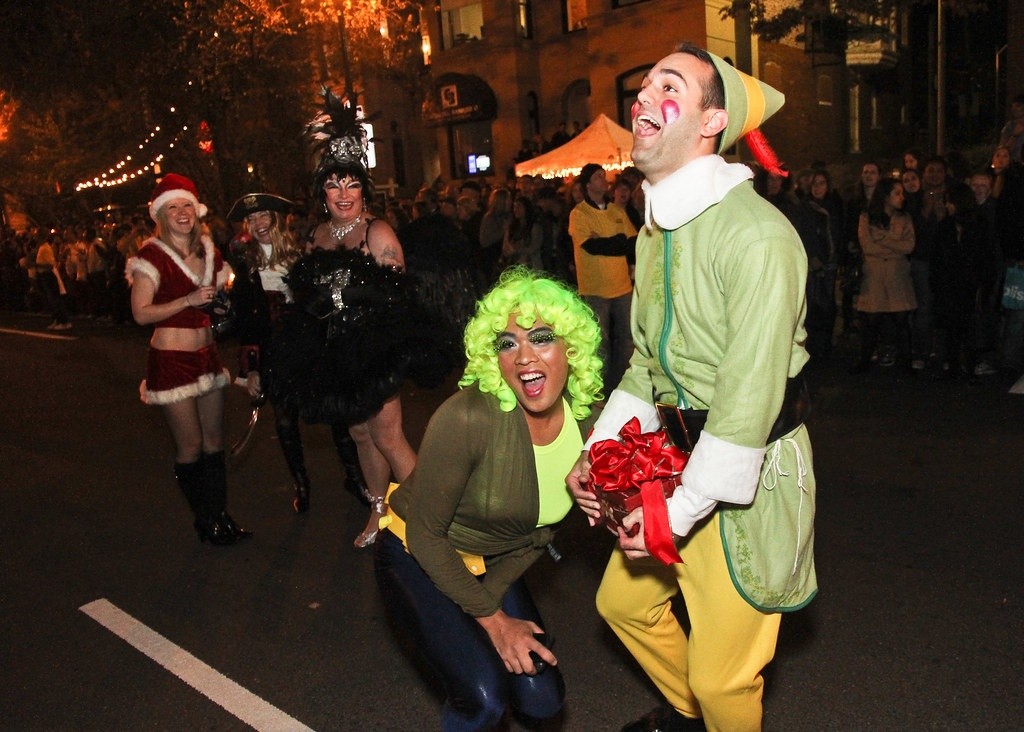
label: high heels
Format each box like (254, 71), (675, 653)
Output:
(353, 501), (387, 552)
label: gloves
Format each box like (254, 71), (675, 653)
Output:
(309, 292), (335, 320)
(331, 303), (371, 327)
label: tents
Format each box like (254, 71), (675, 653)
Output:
(515, 113), (635, 180)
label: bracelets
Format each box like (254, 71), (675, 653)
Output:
(331, 286), (347, 310)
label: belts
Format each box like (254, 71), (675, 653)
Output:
(654, 372), (812, 452)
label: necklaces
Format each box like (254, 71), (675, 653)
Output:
(328, 213), (362, 238)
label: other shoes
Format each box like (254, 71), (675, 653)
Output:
(928, 361), (952, 379)
(48, 319), (57, 329)
(622, 702), (706, 732)
(961, 354), (999, 375)
(55, 322), (73, 330)
(911, 352), (925, 370)
(878, 349), (899, 366)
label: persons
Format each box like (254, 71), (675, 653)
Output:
(566, 163), (638, 379)
(516, 119), (589, 163)
(750, 149), (1024, 376)
(374, 266), (606, 732)
(122, 174), (252, 544)
(227, 193), (377, 513)
(376, 177), (645, 288)
(299, 162), (418, 549)
(567, 42), (819, 732)
(0, 210), (322, 332)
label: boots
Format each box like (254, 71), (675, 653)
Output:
(330, 424), (371, 507)
(202, 450), (255, 537)
(272, 426), (311, 513)
(174, 456), (240, 546)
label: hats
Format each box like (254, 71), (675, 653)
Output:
(310, 86), (371, 194)
(704, 50), (789, 176)
(581, 163), (601, 187)
(227, 171), (296, 220)
(149, 174), (208, 224)
